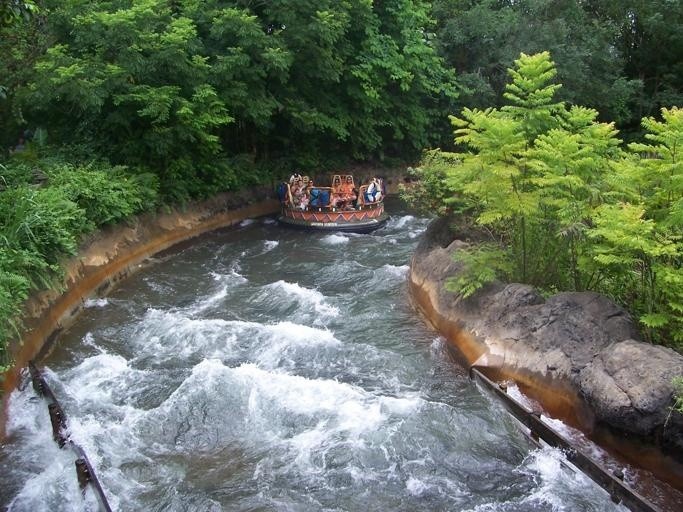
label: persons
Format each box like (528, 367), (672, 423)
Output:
(282, 173), (385, 212)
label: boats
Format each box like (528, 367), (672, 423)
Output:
(275, 174), (390, 234)
